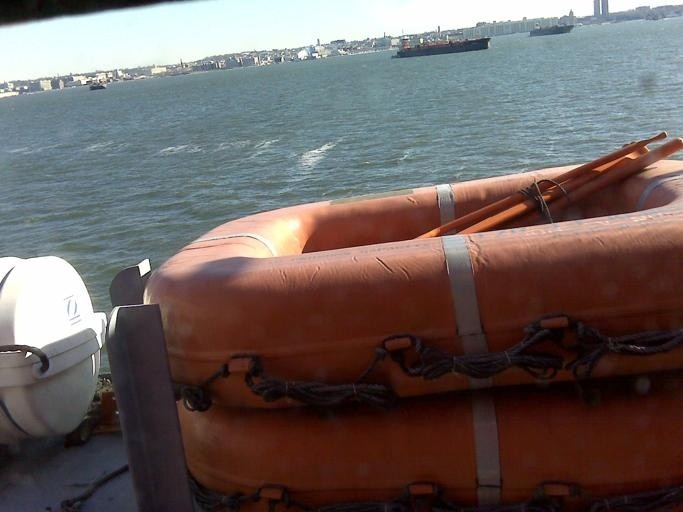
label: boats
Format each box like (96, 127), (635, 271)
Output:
(528, 23), (573, 38)
(90, 80), (106, 91)
(392, 32), (491, 60)
